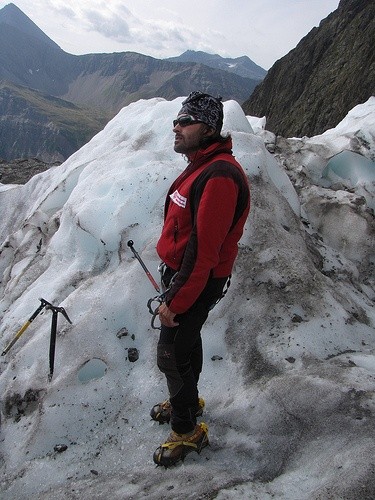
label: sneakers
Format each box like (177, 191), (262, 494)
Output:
(153, 423), (209, 465)
(150, 397), (204, 422)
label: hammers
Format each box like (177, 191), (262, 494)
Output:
(1, 298), (52, 356)
(44, 305), (72, 383)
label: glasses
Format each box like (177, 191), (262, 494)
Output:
(172, 118), (203, 127)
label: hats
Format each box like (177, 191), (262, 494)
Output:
(178, 90), (224, 135)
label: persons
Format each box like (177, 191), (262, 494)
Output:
(150, 89), (252, 467)
